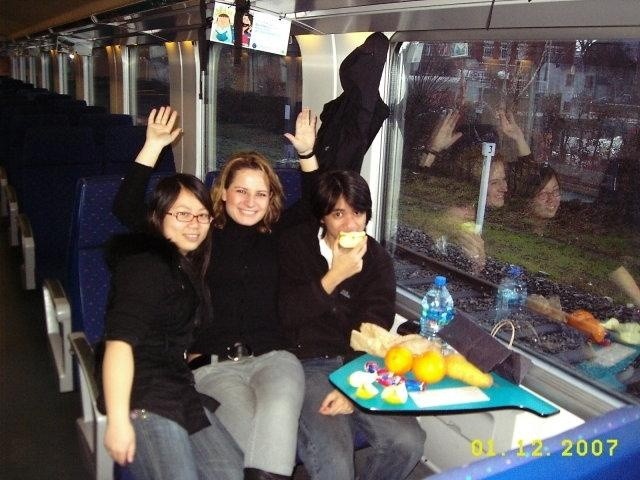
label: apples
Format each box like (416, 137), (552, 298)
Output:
(382, 380), (408, 404)
(614, 321), (640, 345)
(600, 317), (619, 331)
(461, 222), (482, 234)
(338, 230), (368, 249)
(347, 371), (378, 387)
(356, 382), (378, 400)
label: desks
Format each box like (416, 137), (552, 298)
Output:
(328, 334), (559, 418)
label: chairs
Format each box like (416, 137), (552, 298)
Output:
(1, 73), (177, 293)
(70, 175), (160, 480)
(201, 168), (313, 233)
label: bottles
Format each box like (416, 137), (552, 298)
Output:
(493, 264), (528, 327)
(419, 274), (455, 355)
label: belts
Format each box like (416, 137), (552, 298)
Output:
(188, 343), (258, 372)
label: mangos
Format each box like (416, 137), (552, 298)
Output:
(445, 353), (489, 388)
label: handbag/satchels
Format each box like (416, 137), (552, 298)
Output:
(436, 312), (532, 388)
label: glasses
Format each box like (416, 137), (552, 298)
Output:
(537, 189), (562, 200)
(167, 211), (211, 225)
